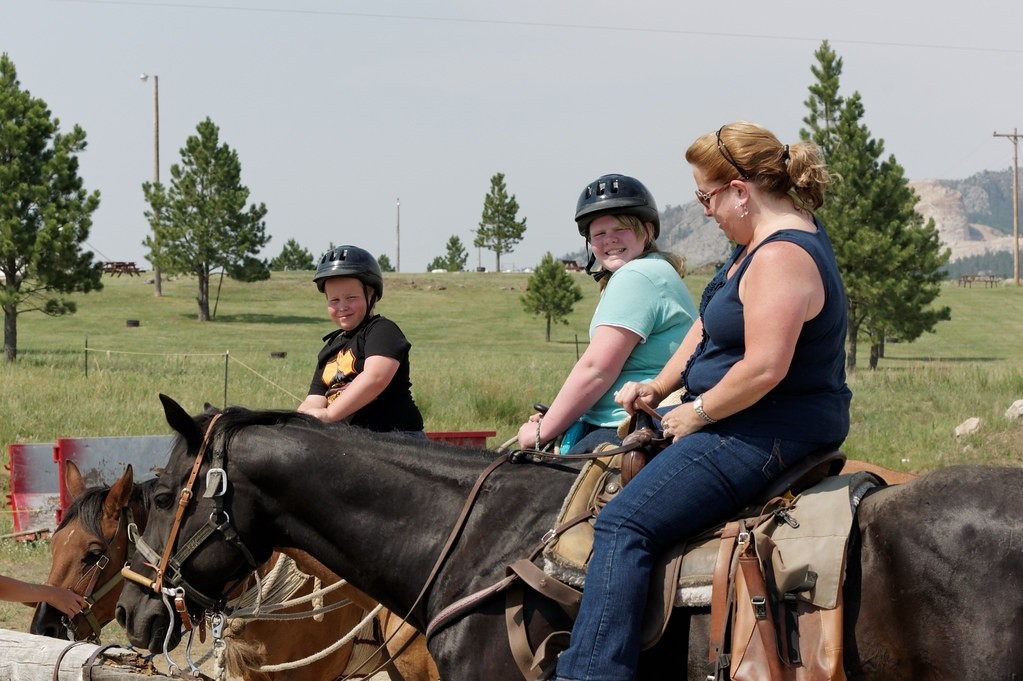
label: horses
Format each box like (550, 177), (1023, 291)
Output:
(28, 458), (924, 681)
(115, 393), (1022, 680)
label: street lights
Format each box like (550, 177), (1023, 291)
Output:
(139, 72), (162, 298)
(396, 198), (400, 273)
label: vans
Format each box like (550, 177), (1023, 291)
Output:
(102, 262), (125, 274)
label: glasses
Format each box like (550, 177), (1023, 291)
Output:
(694, 172), (756, 208)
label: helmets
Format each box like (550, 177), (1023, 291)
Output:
(575, 173), (660, 242)
(312, 245), (383, 302)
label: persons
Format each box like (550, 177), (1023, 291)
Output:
(0, 574), (85, 620)
(555, 123), (853, 681)
(516, 174), (697, 457)
(294, 245), (429, 440)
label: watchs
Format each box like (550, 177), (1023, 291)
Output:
(694, 394), (718, 424)
